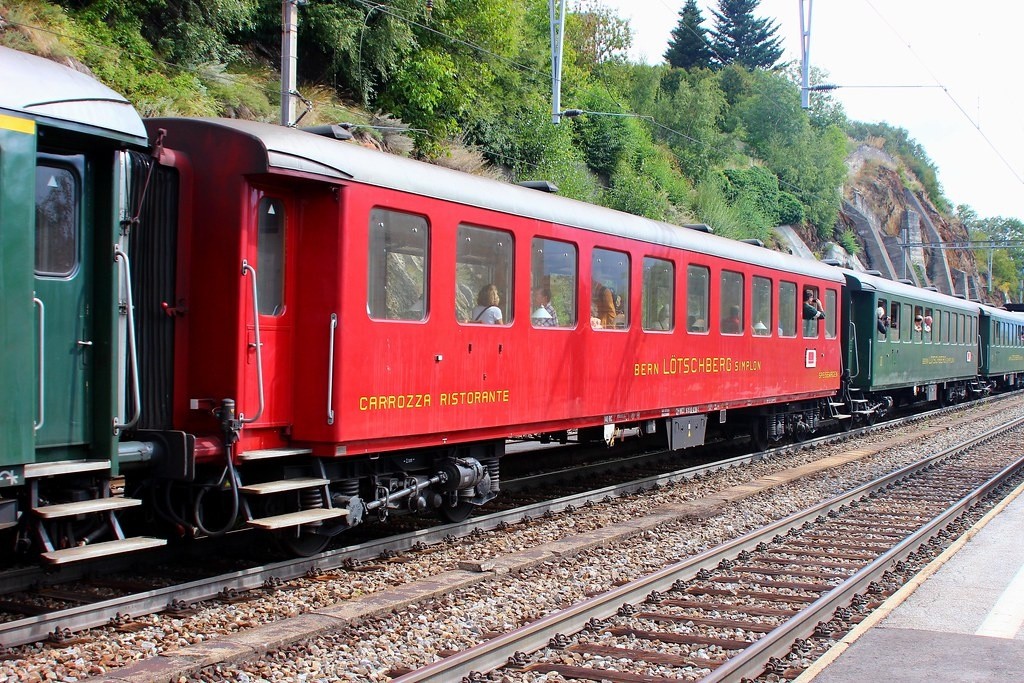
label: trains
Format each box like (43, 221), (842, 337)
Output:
(0, 46), (1024, 565)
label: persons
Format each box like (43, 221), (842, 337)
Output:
(530, 287), (558, 328)
(876, 307), (932, 335)
(591, 267), (618, 330)
(650, 288), (826, 334)
(471, 283), (504, 325)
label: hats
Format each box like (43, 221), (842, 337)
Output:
(691, 319), (705, 327)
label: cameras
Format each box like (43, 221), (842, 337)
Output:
(812, 298), (817, 302)
(884, 316), (889, 320)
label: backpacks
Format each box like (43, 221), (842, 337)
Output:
(599, 286), (624, 315)
